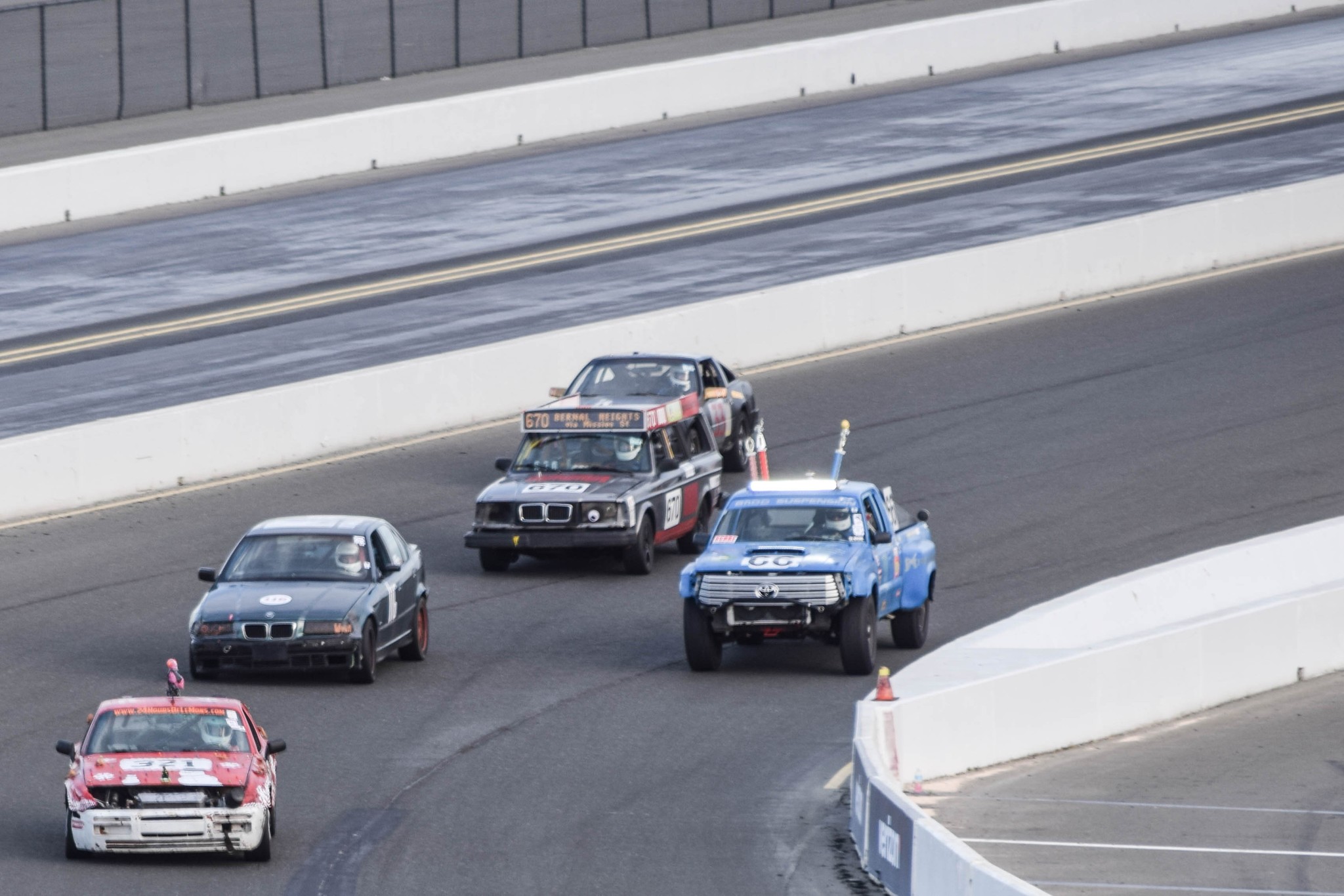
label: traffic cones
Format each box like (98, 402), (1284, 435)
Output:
(869, 665), (899, 701)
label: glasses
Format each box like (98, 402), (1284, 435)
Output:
(829, 512), (845, 518)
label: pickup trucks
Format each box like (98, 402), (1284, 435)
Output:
(678, 476), (939, 674)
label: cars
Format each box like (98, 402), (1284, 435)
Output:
(55, 697), (288, 862)
(189, 515), (430, 685)
(463, 409), (725, 574)
(548, 351), (761, 473)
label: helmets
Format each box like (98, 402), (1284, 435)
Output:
(200, 716), (233, 745)
(614, 438), (641, 460)
(336, 543), (363, 572)
(826, 508), (851, 531)
(670, 361), (693, 386)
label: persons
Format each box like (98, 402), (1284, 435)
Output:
(334, 541), (367, 578)
(658, 366), (697, 398)
(816, 508), (862, 543)
(609, 434), (646, 470)
(198, 715), (241, 753)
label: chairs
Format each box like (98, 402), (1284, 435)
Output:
(570, 439), (596, 467)
(740, 513), (782, 540)
(804, 508), (828, 538)
